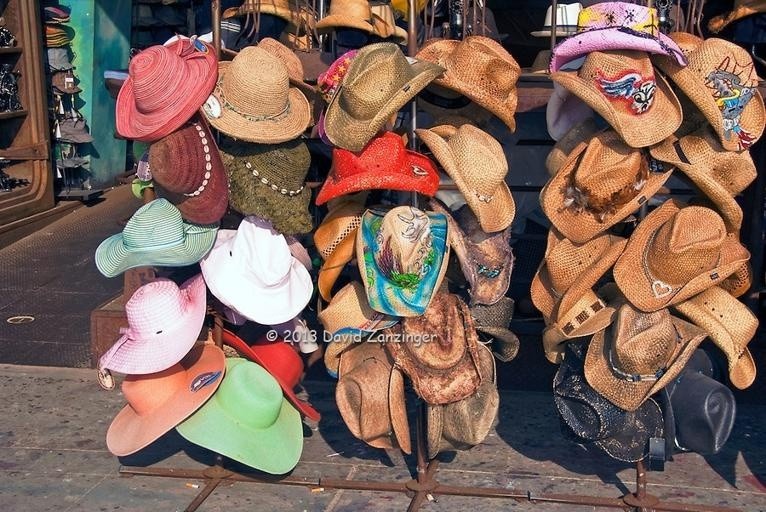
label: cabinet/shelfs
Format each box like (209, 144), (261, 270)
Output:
(0, 0), (59, 229)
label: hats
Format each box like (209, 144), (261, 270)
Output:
(40, 4), (96, 175)
(89, 0), (765, 476)
